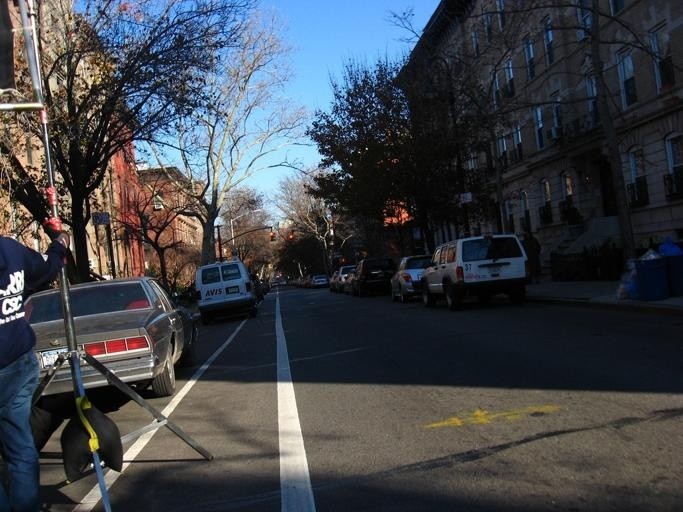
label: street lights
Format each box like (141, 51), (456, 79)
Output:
(230, 208), (263, 246)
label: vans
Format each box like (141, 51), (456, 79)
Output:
(196, 260), (257, 319)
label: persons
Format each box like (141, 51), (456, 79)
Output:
(0, 231), (70, 512)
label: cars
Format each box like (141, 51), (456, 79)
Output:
(22, 276), (199, 397)
(329, 233), (529, 311)
(270, 272), (328, 288)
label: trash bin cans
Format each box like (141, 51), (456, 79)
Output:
(631, 254), (683, 300)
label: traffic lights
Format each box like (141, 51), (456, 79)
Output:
(270, 232), (276, 241)
(289, 232), (294, 240)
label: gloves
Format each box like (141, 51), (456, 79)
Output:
(53, 229), (70, 250)
